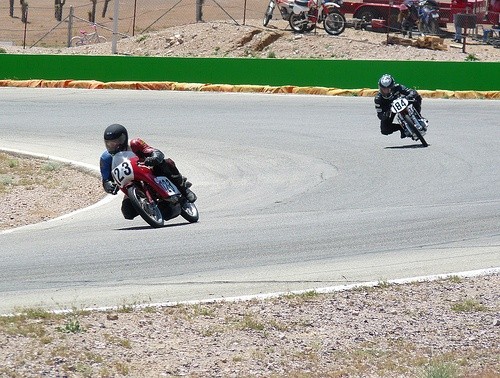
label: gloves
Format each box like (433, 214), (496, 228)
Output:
(145, 157), (159, 166)
(407, 94), (413, 100)
(109, 183), (119, 195)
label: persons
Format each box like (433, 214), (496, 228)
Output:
(398, 0), (468, 43)
(100, 124), (198, 220)
(374, 74), (428, 138)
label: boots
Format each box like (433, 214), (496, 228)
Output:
(171, 173), (196, 203)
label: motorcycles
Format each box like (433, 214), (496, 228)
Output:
(405, 0), (441, 36)
(262, 0), (311, 26)
(109, 151), (199, 228)
(286, 0), (346, 36)
(383, 93), (430, 147)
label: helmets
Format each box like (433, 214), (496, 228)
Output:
(104, 124), (128, 155)
(378, 74), (395, 98)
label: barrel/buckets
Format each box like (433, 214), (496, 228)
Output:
(483, 29), (493, 40)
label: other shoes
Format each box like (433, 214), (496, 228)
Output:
(420, 115), (429, 123)
(400, 124), (406, 138)
